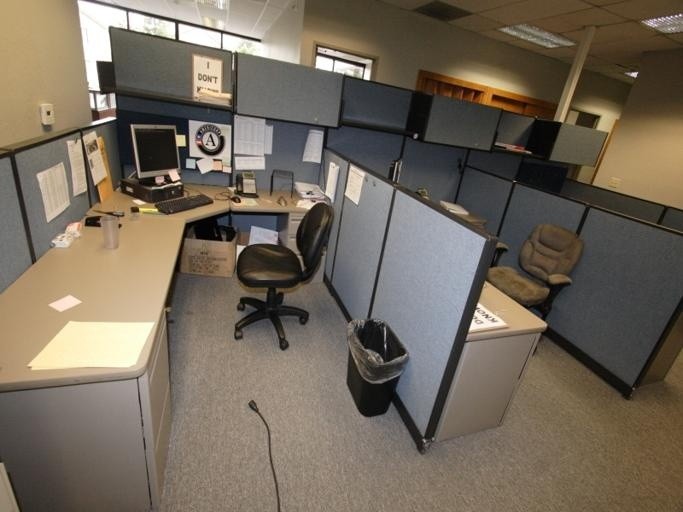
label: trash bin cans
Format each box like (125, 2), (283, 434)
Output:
(346, 317), (409, 417)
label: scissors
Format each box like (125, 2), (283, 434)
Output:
(93, 209), (125, 217)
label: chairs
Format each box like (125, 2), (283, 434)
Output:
(234, 202), (334, 349)
(486, 223), (584, 355)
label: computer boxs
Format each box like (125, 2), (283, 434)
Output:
(120, 176), (184, 203)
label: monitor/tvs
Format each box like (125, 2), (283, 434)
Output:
(130, 123), (182, 187)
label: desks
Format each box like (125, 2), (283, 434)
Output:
(432, 215), (548, 443)
(0, 183), (331, 512)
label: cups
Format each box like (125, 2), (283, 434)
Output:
(100, 214), (120, 250)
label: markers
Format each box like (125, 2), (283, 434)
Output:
(131, 206), (158, 213)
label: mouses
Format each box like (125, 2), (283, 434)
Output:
(230, 195), (241, 203)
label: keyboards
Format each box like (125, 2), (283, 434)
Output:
(155, 193), (214, 214)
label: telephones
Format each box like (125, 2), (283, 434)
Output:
(235, 172), (258, 197)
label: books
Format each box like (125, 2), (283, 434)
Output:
(295, 182), (325, 199)
(495, 142), (532, 154)
(468, 303), (509, 333)
(440, 201), (469, 215)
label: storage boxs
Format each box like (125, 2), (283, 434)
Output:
(180, 226), (239, 277)
